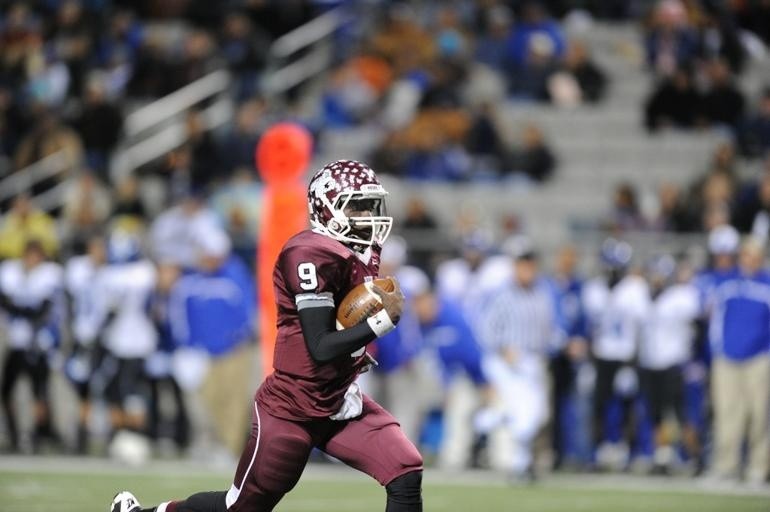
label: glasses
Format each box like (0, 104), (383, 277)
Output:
(350, 201), (375, 210)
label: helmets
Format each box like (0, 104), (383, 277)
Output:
(307, 159), (388, 235)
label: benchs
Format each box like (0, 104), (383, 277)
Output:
(258, 30), (740, 282)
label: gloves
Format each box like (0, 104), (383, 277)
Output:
(329, 383), (362, 420)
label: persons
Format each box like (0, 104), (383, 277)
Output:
(107, 160), (423, 512)
(1, 2), (770, 488)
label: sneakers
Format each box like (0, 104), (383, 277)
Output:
(110, 491), (140, 512)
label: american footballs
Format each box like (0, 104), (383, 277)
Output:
(336, 279), (394, 331)
